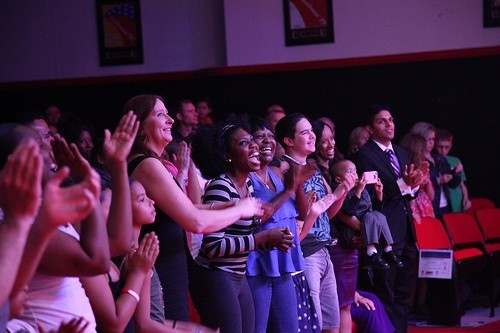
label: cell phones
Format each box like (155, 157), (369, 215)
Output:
(364, 171), (378, 184)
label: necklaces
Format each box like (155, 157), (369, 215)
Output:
(254, 171), (270, 189)
(283, 153), (307, 165)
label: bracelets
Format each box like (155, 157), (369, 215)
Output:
(209, 203), (216, 210)
(172, 320), (178, 328)
(193, 323), (203, 333)
(178, 173), (188, 181)
(122, 288), (140, 303)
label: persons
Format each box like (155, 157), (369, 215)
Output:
(352, 106), (429, 333)
(247, 118), (305, 333)
(123, 95), (263, 323)
(331, 159), (403, 269)
(186, 124), (295, 333)
(269, 113), (340, 333)
(1, 100), (472, 333)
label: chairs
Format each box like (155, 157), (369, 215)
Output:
(413, 196), (500, 325)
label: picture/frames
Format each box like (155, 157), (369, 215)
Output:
(482, 0), (500, 29)
(95, 0), (145, 68)
(282, 0), (335, 47)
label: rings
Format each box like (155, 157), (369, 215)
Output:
(413, 179), (416, 183)
